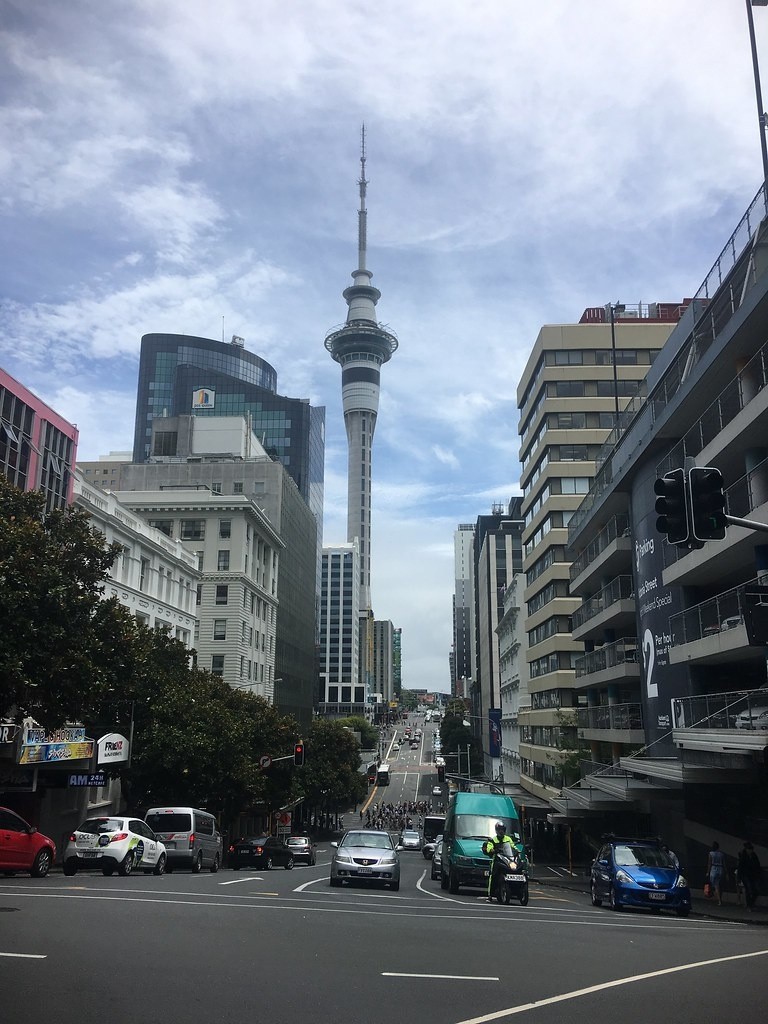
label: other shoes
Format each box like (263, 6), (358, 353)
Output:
(486, 896), (492, 902)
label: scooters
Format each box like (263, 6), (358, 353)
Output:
(491, 849), (530, 907)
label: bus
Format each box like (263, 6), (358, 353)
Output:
(375, 764), (392, 786)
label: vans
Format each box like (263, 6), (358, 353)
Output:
(423, 792), (528, 893)
(145, 807), (224, 872)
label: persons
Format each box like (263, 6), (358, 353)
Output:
(486, 822), (521, 903)
(705, 841), (727, 906)
(656, 845), (679, 869)
(735, 842), (762, 912)
(359, 800), (444, 829)
(314, 815), (343, 830)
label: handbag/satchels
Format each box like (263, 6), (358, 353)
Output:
(704, 876), (714, 898)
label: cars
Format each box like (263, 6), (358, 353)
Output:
(329, 829), (404, 891)
(227, 836), (295, 871)
(409, 729), (423, 751)
(285, 837), (318, 865)
(398, 829), (423, 851)
(432, 786), (443, 796)
(62, 817), (169, 876)
(392, 743), (400, 751)
(708, 684), (768, 730)
(591, 842), (692, 918)
(0, 807), (56, 877)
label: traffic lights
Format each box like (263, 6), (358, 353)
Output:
(688, 468), (726, 540)
(655, 469), (689, 547)
(294, 744), (303, 767)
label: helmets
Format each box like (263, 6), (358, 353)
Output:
(495, 821), (506, 833)
